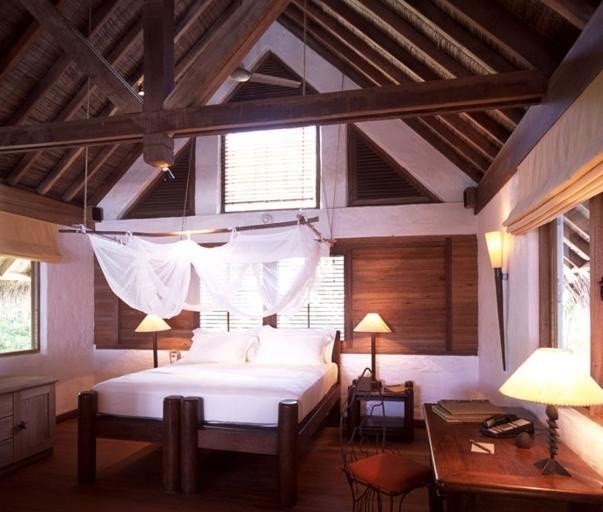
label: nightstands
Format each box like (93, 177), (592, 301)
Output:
(347, 380), (415, 441)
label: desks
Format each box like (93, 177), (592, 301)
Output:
(421, 401), (603, 511)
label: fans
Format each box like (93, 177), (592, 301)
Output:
(227, 61), (302, 91)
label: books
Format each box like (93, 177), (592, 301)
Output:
(432, 399), (507, 423)
(383, 383), (407, 393)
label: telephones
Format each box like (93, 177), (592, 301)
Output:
(481, 414), (534, 438)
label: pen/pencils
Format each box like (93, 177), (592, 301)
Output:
(469, 439), (492, 455)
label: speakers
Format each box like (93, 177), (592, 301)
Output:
(463, 187), (476, 209)
(87, 205), (101, 223)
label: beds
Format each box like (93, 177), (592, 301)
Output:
(76, 325), (342, 507)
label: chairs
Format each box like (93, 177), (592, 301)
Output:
(335, 367), (435, 512)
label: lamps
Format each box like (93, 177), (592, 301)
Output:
(134, 314), (171, 368)
(484, 230), (507, 371)
(497, 347), (602, 477)
(351, 311), (392, 379)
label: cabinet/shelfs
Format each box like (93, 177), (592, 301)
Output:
(0, 374), (60, 479)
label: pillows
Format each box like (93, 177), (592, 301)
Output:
(175, 325), (336, 366)
